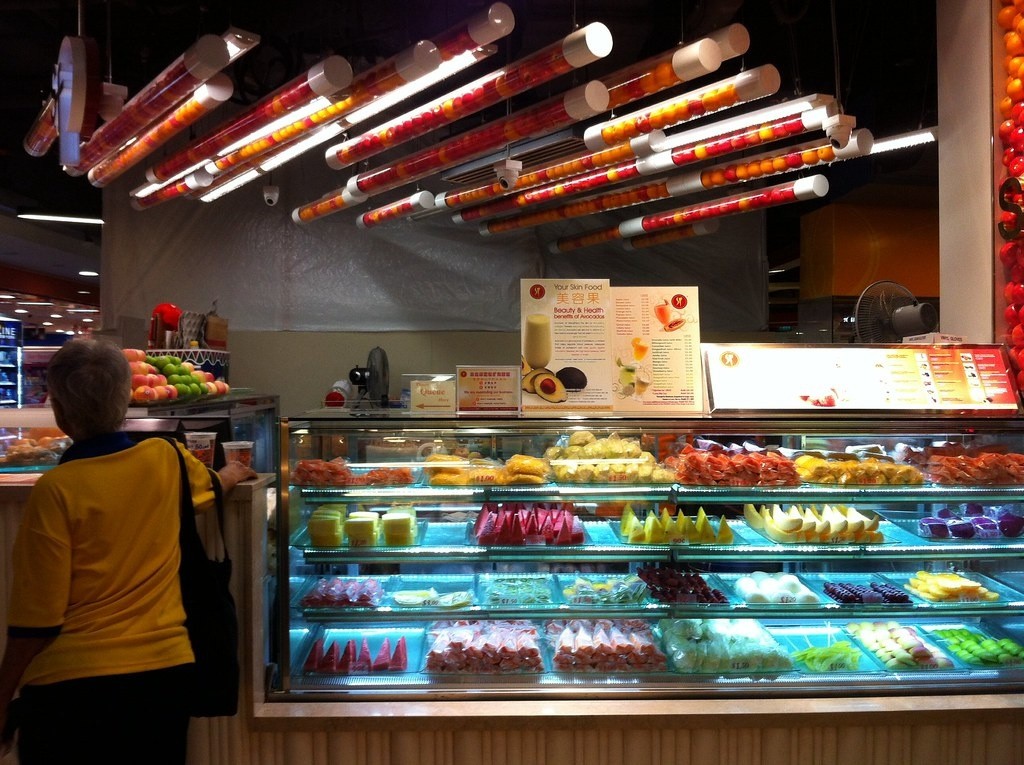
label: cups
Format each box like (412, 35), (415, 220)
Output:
(525, 315), (551, 369)
(185, 433), (217, 469)
(221, 442), (254, 467)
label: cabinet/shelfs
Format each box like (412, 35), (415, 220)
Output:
(798, 204), (940, 304)
(0, 318), (75, 459)
(271, 408), (1023, 697)
(0, 387), (285, 692)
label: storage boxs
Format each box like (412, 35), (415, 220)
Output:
(141, 348), (230, 387)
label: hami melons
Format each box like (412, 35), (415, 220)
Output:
(743, 502), (883, 543)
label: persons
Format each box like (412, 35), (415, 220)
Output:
(0, 340), (259, 765)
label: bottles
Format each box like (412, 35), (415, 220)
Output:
(189, 341), (202, 367)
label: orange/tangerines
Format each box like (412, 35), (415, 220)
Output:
(997, 0), (1024, 402)
(90, 59), (831, 227)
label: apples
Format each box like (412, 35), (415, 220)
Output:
(119, 348), (231, 401)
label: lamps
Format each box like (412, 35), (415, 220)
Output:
(15, 204), (106, 224)
(200, 43), (498, 203)
(117, 29), (263, 150)
(596, 96), (940, 213)
(127, 86), (353, 201)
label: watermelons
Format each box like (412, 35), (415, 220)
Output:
(474, 503), (585, 545)
(301, 637), (407, 672)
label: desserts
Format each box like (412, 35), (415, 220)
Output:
(307, 505), (414, 546)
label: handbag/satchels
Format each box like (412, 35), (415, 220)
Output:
(163, 436), (239, 717)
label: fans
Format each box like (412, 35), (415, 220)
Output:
(849, 279), (932, 341)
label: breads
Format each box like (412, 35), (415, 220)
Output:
(0, 427), (74, 466)
(794, 455), (923, 485)
(423, 430), (678, 484)
(652, 619), (794, 672)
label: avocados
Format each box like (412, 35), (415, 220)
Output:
(520, 356), (588, 404)
